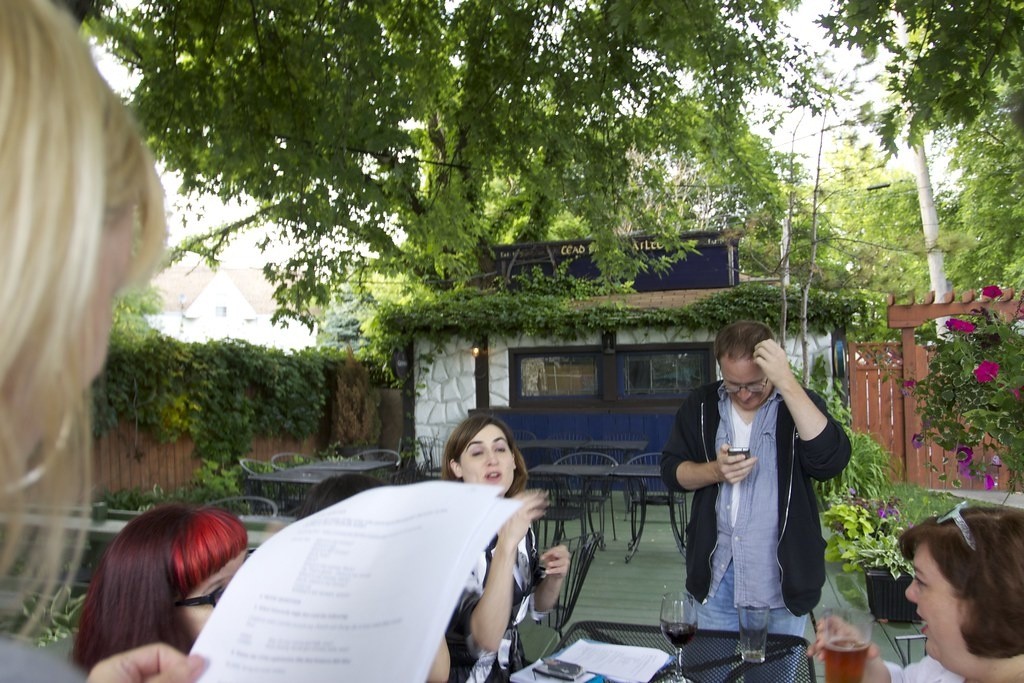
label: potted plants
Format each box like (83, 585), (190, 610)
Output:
(832, 499), (923, 624)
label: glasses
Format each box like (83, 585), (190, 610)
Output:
(173, 549), (256, 607)
(719, 369), (768, 392)
(936, 501), (976, 551)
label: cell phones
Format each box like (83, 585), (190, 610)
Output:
(726, 447), (750, 460)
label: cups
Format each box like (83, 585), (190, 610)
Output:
(824, 608), (872, 683)
(737, 601), (770, 662)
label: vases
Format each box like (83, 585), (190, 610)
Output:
(842, 525), (886, 559)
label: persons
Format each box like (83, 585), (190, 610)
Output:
(806, 503), (1024, 683)
(440, 413), (572, 683)
(0, 0), (169, 683)
(659, 321), (852, 683)
(69, 474), (449, 683)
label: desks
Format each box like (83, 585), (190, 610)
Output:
(300, 457), (394, 499)
(247, 469), (335, 523)
(526, 464), (616, 555)
(548, 621), (817, 683)
(294, 461), (348, 510)
(614, 463), (688, 565)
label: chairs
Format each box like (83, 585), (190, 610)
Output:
(622, 452), (687, 564)
(240, 458), (298, 512)
(271, 453), (320, 503)
(350, 450), (402, 485)
(536, 533), (604, 637)
(205, 495), (279, 521)
(417, 435), (445, 480)
(549, 452), (617, 551)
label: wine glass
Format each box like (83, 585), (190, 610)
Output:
(661, 592), (698, 683)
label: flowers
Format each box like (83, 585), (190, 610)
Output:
(829, 497), (913, 536)
